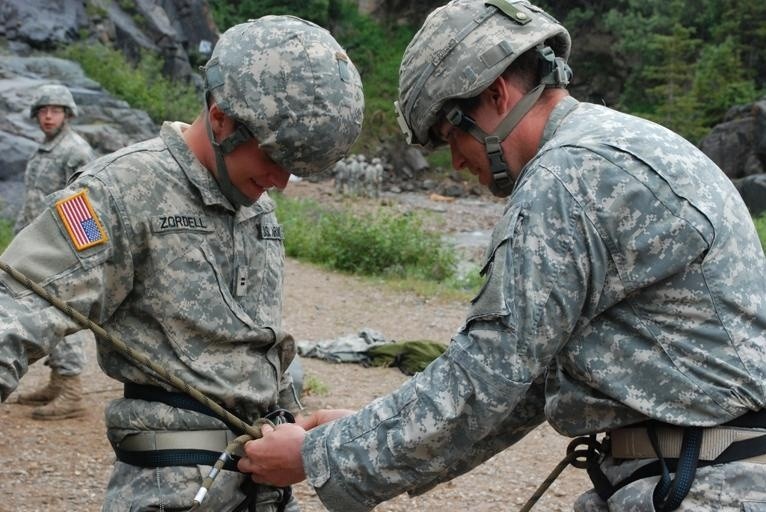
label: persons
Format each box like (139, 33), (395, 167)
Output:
(331, 152), (384, 202)
(13, 83), (96, 421)
(235, 0), (766, 511)
(0, 12), (366, 512)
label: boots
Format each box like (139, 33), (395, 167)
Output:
(16, 374), (59, 406)
(35, 374), (86, 420)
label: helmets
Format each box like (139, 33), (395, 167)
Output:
(201, 12), (364, 186)
(391, 1), (575, 146)
(29, 83), (80, 118)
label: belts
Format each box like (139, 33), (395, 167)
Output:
(609, 424), (765, 467)
(113, 425), (251, 460)
